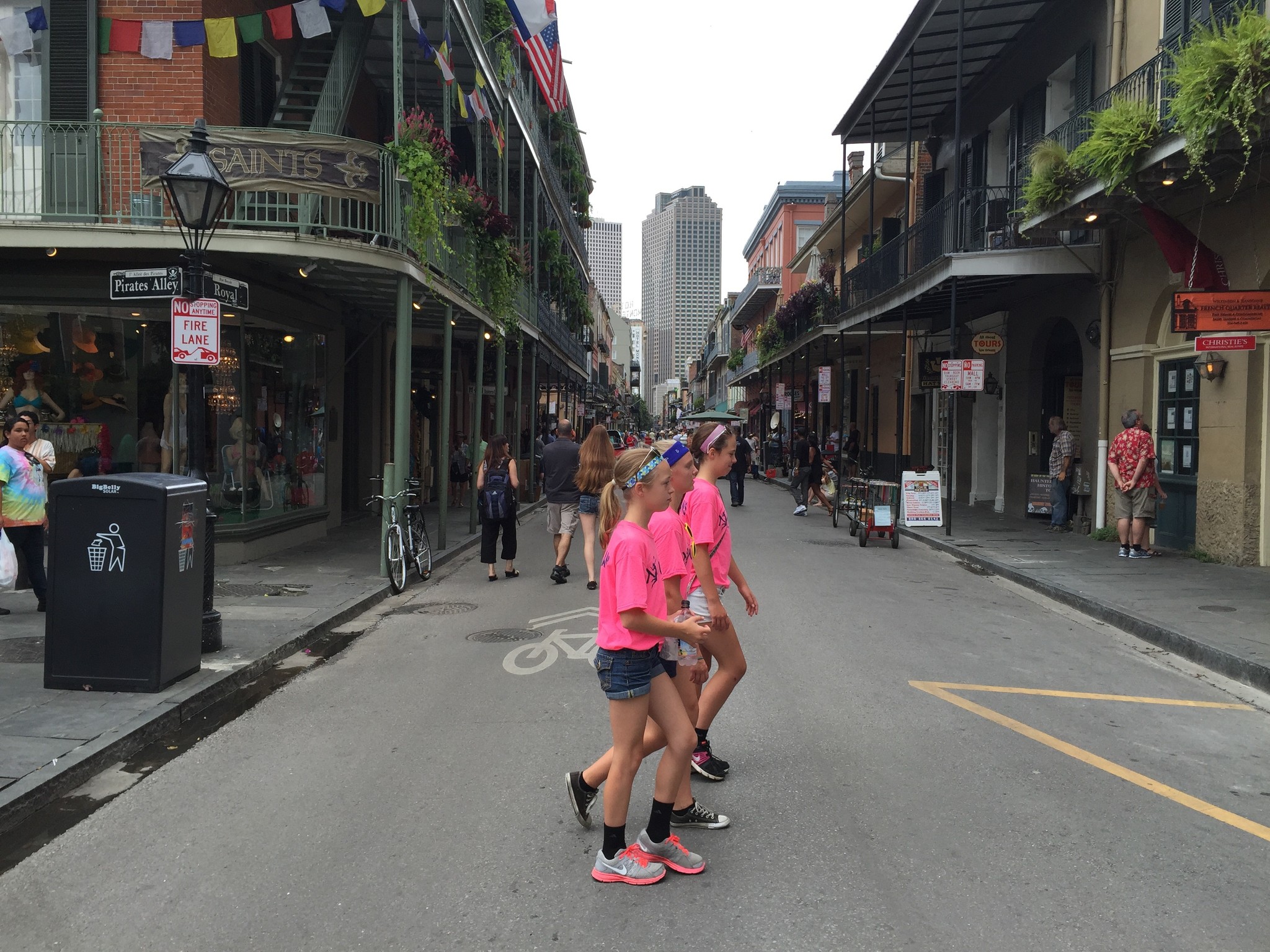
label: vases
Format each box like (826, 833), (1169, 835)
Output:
(395, 164), (413, 195)
(445, 211), (466, 233)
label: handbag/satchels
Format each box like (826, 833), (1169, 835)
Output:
(847, 441), (859, 454)
(0, 526), (18, 591)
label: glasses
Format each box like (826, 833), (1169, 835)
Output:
(707, 423), (732, 456)
(635, 446), (662, 483)
(24, 452), (40, 465)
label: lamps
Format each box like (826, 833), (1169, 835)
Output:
(451, 311), (460, 325)
(485, 329), (498, 339)
(983, 372), (1002, 401)
(831, 336), (838, 342)
(776, 358), (790, 370)
(1162, 172), (1178, 186)
(821, 249), (835, 265)
(1194, 351), (1228, 382)
(413, 292), (429, 309)
(45, 248), (56, 257)
(960, 390), (976, 403)
(798, 350), (805, 359)
(299, 260), (318, 277)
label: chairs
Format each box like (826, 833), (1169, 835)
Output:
(973, 198), (1017, 251)
(219, 445), (274, 510)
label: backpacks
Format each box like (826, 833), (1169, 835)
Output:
(267, 450), (319, 512)
(477, 456), (521, 526)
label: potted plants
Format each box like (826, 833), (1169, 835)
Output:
(552, 142), (584, 171)
(570, 190), (592, 212)
(551, 112), (564, 141)
(579, 214), (595, 228)
(561, 169), (585, 193)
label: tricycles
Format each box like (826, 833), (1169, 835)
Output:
(831, 459), (876, 528)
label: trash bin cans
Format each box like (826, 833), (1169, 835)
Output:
(44, 472), (208, 694)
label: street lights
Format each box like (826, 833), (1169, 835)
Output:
(758, 386), (770, 442)
(160, 117), (233, 650)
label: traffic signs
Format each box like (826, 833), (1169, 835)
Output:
(818, 384), (831, 403)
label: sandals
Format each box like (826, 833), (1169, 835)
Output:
(827, 506), (834, 516)
(1146, 548), (1162, 556)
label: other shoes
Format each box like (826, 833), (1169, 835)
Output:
(451, 497), (464, 507)
(731, 502), (742, 506)
(488, 564), (598, 590)
(36, 602), (46, 612)
(0, 608), (11, 615)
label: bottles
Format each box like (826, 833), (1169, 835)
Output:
(678, 600), (697, 665)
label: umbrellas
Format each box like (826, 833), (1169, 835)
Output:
(678, 409), (746, 422)
(804, 243), (830, 294)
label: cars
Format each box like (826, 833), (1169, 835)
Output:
(677, 434), (688, 445)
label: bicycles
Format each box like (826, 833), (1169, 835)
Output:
(362, 475), (432, 595)
(281, 460), (312, 513)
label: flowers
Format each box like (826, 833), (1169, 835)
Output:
(409, 105), (454, 157)
(447, 169), (494, 231)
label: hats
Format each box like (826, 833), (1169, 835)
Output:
(10, 328), (131, 414)
(668, 431), (674, 435)
(453, 431), (468, 442)
(659, 430), (666, 434)
(630, 432), (633, 434)
(473, 429), (483, 438)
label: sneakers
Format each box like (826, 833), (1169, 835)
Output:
(591, 843), (666, 885)
(669, 795), (731, 829)
(632, 828), (706, 874)
(1118, 546), (1152, 559)
(564, 771), (600, 827)
(1044, 522), (1069, 533)
(690, 738), (730, 780)
(793, 504), (808, 516)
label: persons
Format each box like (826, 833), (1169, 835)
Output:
(592, 446), (712, 887)
(448, 419), (861, 590)
(476, 434), (520, 581)
(677, 423), (758, 780)
(564, 440), (730, 831)
(0, 410), (57, 614)
(0, 361), (66, 423)
(1107, 409), (1168, 558)
(68, 445), (104, 479)
(226, 417), (271, 502)
(161, 373), (188, 475)
(1044, 416), (1076, 533)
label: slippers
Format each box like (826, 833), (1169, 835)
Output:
(844, 477), (851, 481)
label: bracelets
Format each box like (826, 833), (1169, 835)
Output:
(748, 465), (752, 467)
(795, 468), (799, 470)
(832, 467), (834, 470)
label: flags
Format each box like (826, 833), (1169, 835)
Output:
(614, 388), (619, 399)
(740, 324), (754, 350)
(512, 21), (568, 114)
(504, 0), (558, 42)
(676, 407), (683, 422)
